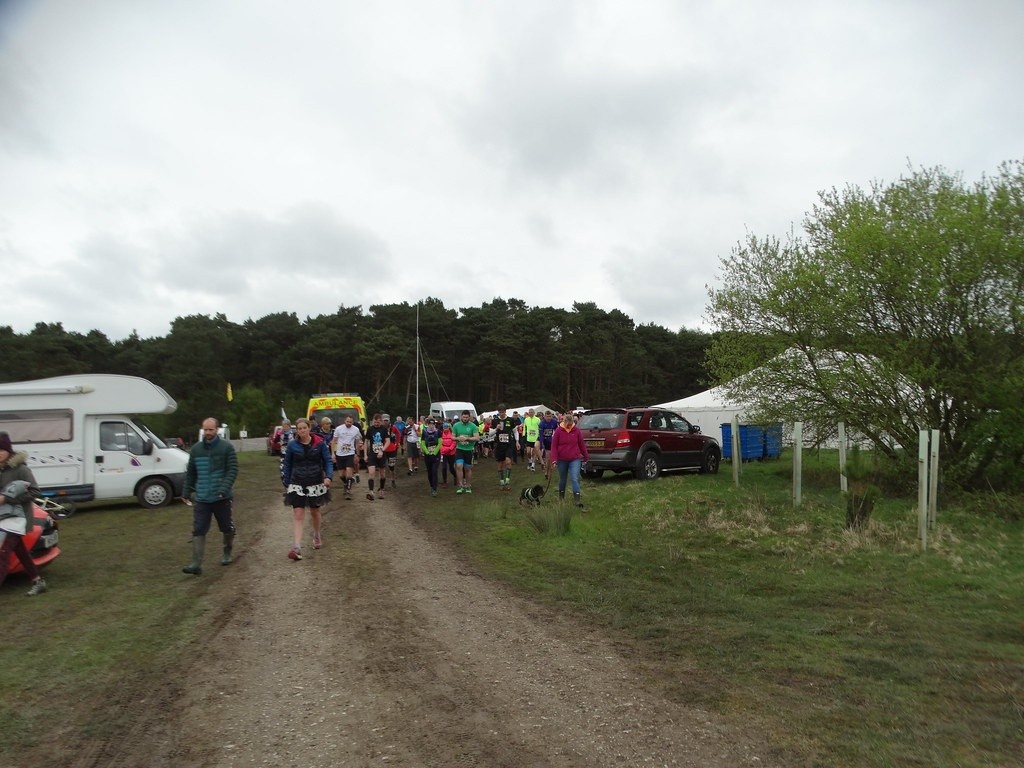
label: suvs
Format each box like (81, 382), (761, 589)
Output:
(573, 406), (722, 482)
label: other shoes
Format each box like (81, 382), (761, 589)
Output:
(344, 493), (351, 500)
(355, 475), (360, 483)
(343, 488), (347, 494)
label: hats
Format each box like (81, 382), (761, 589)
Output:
(443, 423), (448, 426)
(453, 415), (458, 420)
(382, 414), (390, 421)
(0, 431), (13, 453)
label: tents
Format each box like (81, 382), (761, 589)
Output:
(478, 404), (555, 418)
(649, 343), (962, 451)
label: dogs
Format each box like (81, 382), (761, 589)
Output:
(519, 484), (545, 508)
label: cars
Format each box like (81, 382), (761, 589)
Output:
(266, 425), (298, 456)
(113, 433), (186, 454)
(0, 500), (62, 574)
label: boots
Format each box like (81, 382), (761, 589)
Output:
(221, 534), (234, 564)
(573, 492), (583, 508)
(559, 490), (565, 501)
(182, 536), (205, 574)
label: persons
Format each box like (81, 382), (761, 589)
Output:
(309, 404), (584, 501)
(551, 415), (589, 507)
(283, 418), (333, 560)
(0, 432), (47, 596)
(273, 419), (296, 484)
(182, 418), (239, 574)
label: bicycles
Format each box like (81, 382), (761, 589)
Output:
(32, 491), (74, 519)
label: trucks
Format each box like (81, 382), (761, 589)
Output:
(307, 392), (369, 437)
(0, 374), (195, 507)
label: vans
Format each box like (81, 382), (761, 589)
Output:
(429, 402), (478, 422)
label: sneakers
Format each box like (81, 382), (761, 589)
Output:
(287, 548), (302, 560)
(25, 579), (47, 595)
(366, 453), (547, 501)
(312, 536), (321, 549)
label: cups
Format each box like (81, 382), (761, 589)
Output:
(500, 422), (503, 430)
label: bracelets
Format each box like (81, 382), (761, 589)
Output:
(382, 450), (384, 452)
